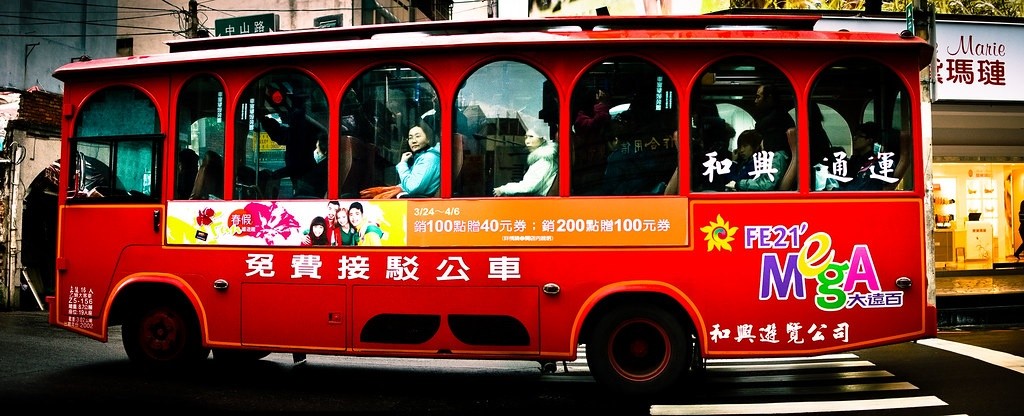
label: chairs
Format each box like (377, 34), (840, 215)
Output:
(175, 120), (909, 199)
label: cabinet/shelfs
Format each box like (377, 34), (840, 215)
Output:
(968, 176), (998, 236)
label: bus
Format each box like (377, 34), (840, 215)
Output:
(44, 13), (937, 404)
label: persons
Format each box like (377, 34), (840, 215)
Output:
(493, 124), (559, 197)
(258, 107), (322, 198)
(575, 86), (675, 194)
(300, 201), (384, 246)
(692, 82), (797, 192)
(815, 101), (910, 189)
(131, 149), (199, 201)
(395, 121), (440, 198)
(1013, 200), (1024, 259)
